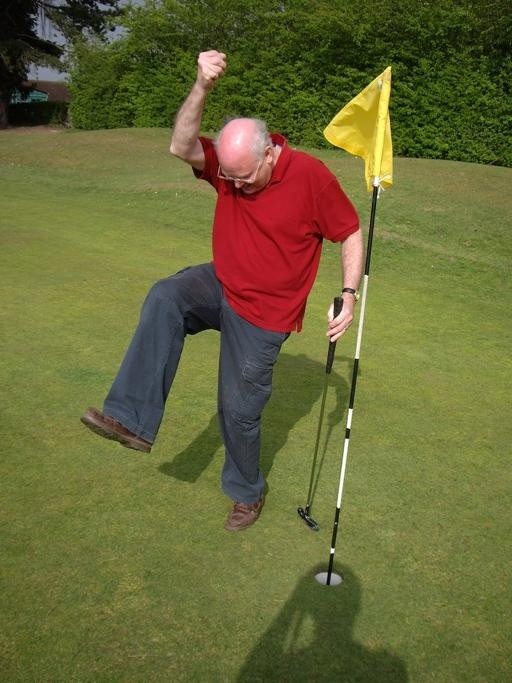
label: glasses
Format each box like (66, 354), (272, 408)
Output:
(216, 153), (266, 184)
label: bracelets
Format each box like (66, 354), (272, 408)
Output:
(338, 285), (364, 303)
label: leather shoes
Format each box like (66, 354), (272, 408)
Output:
(80, 402), (156, 454)
(224, 480), (269, 531)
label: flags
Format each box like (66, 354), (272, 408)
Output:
(323, 64), (396, 188)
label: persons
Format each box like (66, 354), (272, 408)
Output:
(84, 49), (365, 534)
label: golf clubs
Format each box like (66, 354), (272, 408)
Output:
(299, 297), (345, 532)
